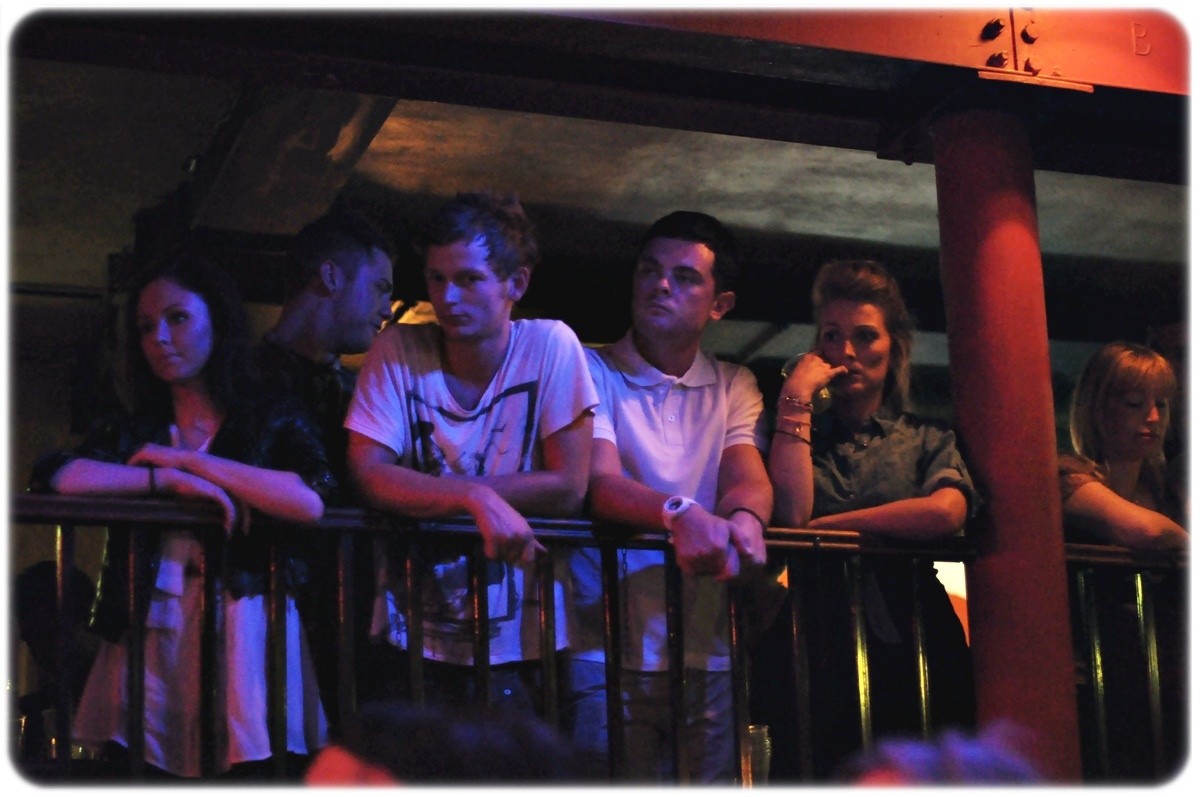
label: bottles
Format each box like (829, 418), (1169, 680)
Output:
(749, 725), (771, 785)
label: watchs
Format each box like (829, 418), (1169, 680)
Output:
(662, 496), (697, 543)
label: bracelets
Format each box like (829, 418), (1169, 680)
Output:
(775, 398), (815, 446)
(728, 507), (767, 535)
(150, 463), (157, 495)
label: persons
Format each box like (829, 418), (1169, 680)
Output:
(343, 200), (601, 714)
(1060, 345), (1188, 786)
(32, 213), (394, 781)
(565, 212), (774, 781)
(854, 732), (1033, 786)
(770, 264), (986, 781)
(303, 705), (576, 785)
